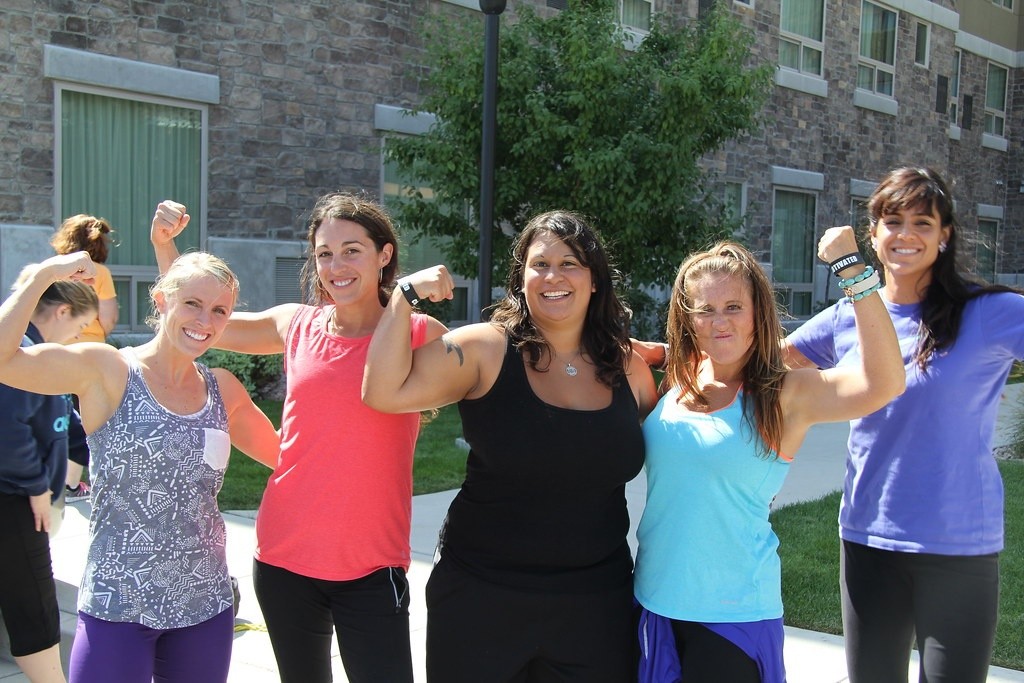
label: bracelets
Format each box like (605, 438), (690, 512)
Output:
(651, 343), (670, 374)
(827, 252), (867, 279)
(836, 266), (882, 303)
(395, 274), (420, 307)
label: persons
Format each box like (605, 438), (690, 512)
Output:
(629, 164), (1023, 682)
(362, 212), (657, 683)
(52, 215), (117, 502)
(148, 192), (451, 683)
(0, 279), (99, 683)
(0, 248), (281, 683)
(634, 224), (907, 683)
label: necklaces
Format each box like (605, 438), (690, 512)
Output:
(551, 345), (583, 378)
